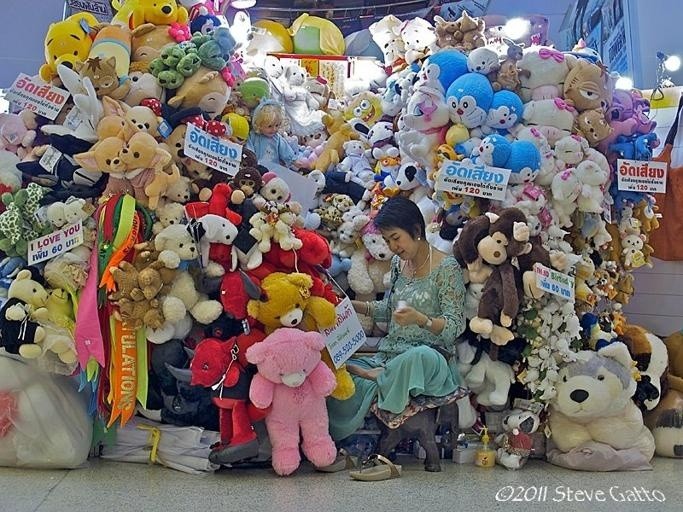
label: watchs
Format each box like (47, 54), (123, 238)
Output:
(424, 317), (433, 332)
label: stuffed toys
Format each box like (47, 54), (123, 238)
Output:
(36, 288), (78, 363)
(392, 16), (440, 66)
(634, 199), (659, 231)
(0, 148), (22, 213)
(349, 114), (399, 159)
(303, 169), (326, 192)
(190, 336), (260, 465)
(16, 133), (110, 204)
(62, 196), (97, 262)
(168, 66), (235, 120)
(584, 148), (610, 176)
(383, 39), (407, 75)
(0, 355), (94, 470)
(265, 228), (332, 274)
(0, 270), (49, 359)
(152, 202), (188, 235)
(369, 15), (403, 52)
(0, 109), (38, 160)
(419, 48), (470, 97)
(617, 324), (669, 414)
(245, 328), (337, 476)
(73, 128), (135, 198)
(518, 302), (580, 367)
(301, 140), (327, 170)
(129, 23), (190, 74)
(620, 232), (646, 268)
(327, 255), (352, 277)
(551, 167), (582, 227)
(461, 134), (510, 167)
(222, 0), (257, 53)
(162, 180), (199, 205)
(380, 77), (403, 116)
(123, 61), (233, 139)
(186, 214), (262, 272)
(44, 252), (91, 292)
(113, 298), (164, 330)
(119, 117), (180, 210)
(616, 277), (634, 305)
(87, 95), (125, 152)
(229, 167), (265, 231)
(329, 212), (359, 258)
(477, 90), (523, 140)
(454, 9), (487, 50)
(75, 24), (133, 100)
(252, 20), (293, 54)
(434, 15), (460, 47)
(630, 89), (656, 134)
(235, 77), (271, 122)
(347, 214), (396, 335)
(521, 97), (577, 148)
(337, 140), (376, 190)
(41, 64), (104, 143)
(237, 329), (272, 421)
(399, 87), (450, 170)
(0, 257), (48, 289)
(346, 92), (382, 126)
(514, 346), (558, 415)
(609, 133), (660, 208)
(546, 342), (656, 472)
(580, 311), (617, 351)
(644, 329), (683, 458)
(517, 124), (564, 185)
(0, 190), (28, 257)
(506, 140), (541, 185)
(7, 182), (52, 256)
(563, 38), (603, 66)
(279, 64), (328, 137)
(576, 160), (608, 213)
(288, 13), (346, 55)
(111, 0), (189, 30)
(193, 149), (258, 189)
(254, 54), (297, 137)
(511, 232), (550, 300)
(145, 312), (192, 344)
(552, 229), (620, 311)
(619, 203), (641, 239)
(468, 47), (501, 82)
(107, 260), (165, 301)
(563, 56), (617, 113)
(148, 27), (236, 90)
(273, 213), (303, 251)
(396, 161), (435, 228)
(247, 272), (356, 401)
(249, 172), (292, 237)
(493, 411), (540, 470)
(528, 207), (552, 236)
(165, 124), (213, 179)
(610, 302), (628, 332)
(40, 11), (99, 82)
(190, 6), (222, 36)
(317, 115), (360, 173)
(437, 207), (463, 241)
(139, 339), (220, 431)
(46, 202), (68, 232)
(641, 232), (655, 269)
(465, 283), (484, 320)
(344, 28), (385, 62)
(118, 100), (163, 137)
(597, 88), (638, 154)
(453, 208), (529, 284)
(445, 72), (494, 157)
(135, 250), (175, 285)
(394, 72), (418, 107)
(469, 236), (552, 345)
(491, 38), (531, 97)
(312, 194), (355, 230)
(442, 191), (464, 212)
(489, 185), (550, 208)
(572, 107), (615, 148)
(154, 224), (225, 324)
(374, 157), (401, 198)
(304, 72), (330, 108)
(555, 134), (584, 171)
(456, 340), (516, 406)
(516, 44), (577, 103)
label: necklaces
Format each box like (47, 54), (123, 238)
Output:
(408, 249), (430, 278)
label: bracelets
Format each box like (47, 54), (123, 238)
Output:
(365, 301), (369, 316)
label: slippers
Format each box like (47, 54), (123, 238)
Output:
(316, 448), (360, 472)
(351, 453), (402, 481)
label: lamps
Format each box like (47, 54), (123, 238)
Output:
(654, 50), (680, 89)
(500, 17), (531, 39)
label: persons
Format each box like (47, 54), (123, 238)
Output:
(327, 194), (466, 443)
(244, 99), (304, 169)
